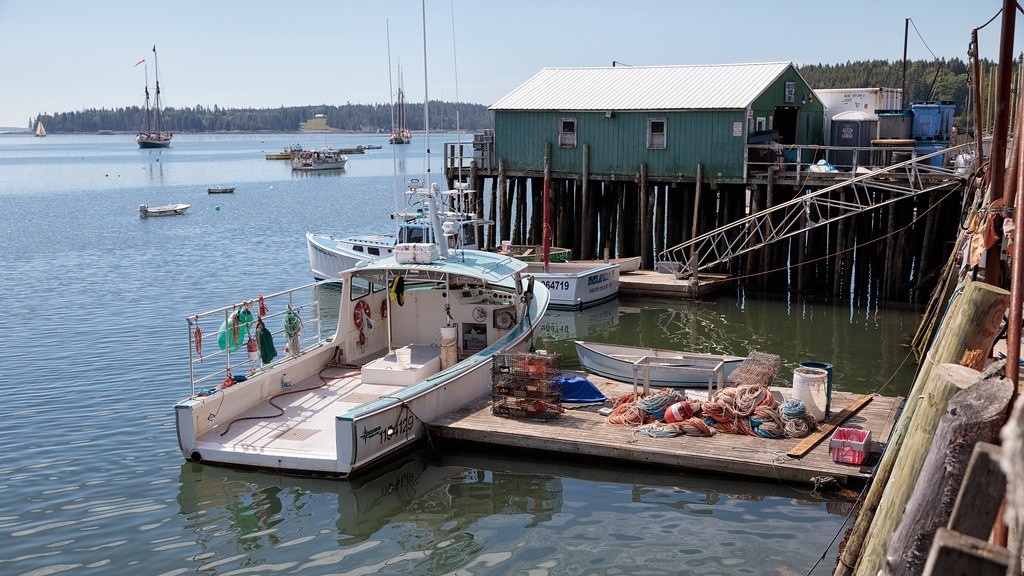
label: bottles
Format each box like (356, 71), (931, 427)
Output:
(283, 374), (292, 389)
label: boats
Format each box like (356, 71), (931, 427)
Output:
(138, 202), (191, 218)
(485, 243), (572, 263)
(572, 339), (790, 390)
(560, 254), (643, 273)
(167, 0), (554, 481)
(546, 373), (609, 404)
(206, 185), (237, 195)
(262, 111), (382, 170)
(302, 178), (622, 313)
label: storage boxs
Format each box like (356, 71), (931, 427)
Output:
(829, 427), (872, 464)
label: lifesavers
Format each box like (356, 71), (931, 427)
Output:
(353, 300), (372, 328)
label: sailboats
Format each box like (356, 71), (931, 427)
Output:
(34, 121), (47, 138)
(133, 44), (176, 148)
(389, 57), (414, 145)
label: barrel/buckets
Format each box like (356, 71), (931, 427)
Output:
(800, 360), (833, 414)
(396, 347), (412, 369)
(792, 368), (828, 422)
